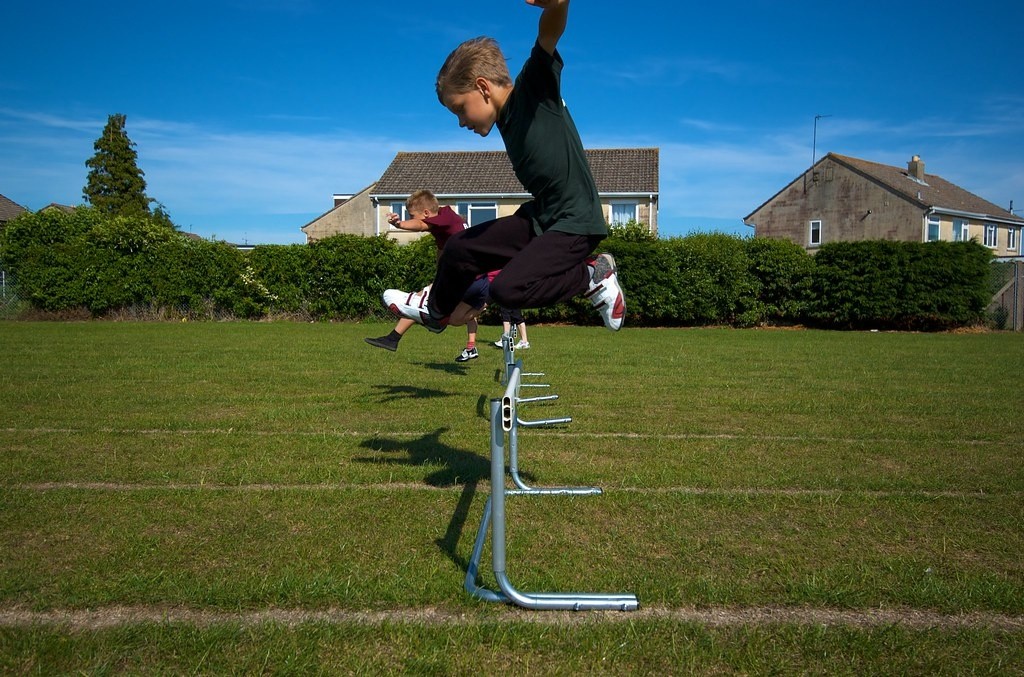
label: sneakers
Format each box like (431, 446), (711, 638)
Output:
(583, 252), (626, 332)
(455, 347), (479, 362)
(494, 338), (503, 347)
(364, 336), (398, 352)
(383, 286), (450, 334)
(514, 340), (530, 349)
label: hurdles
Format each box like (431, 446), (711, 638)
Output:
(463, 323), (640, 612)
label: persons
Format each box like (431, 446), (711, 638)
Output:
(384, 0), (627, 331)
(364, 189), (530, 362)
